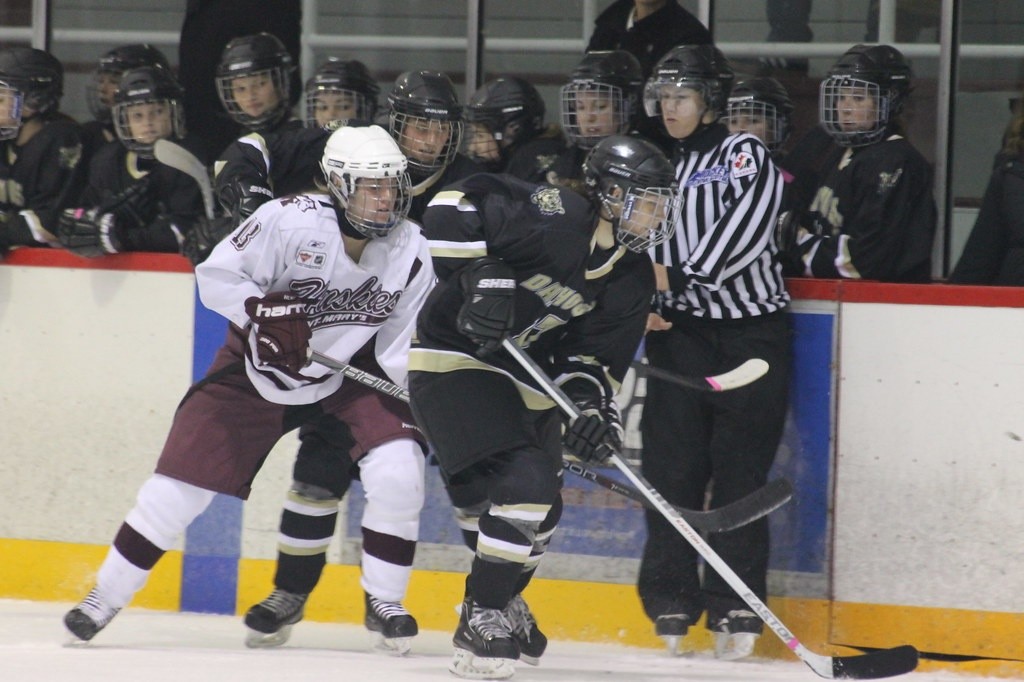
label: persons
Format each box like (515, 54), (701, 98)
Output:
(408, 133), (683, 658)
(559, 0), (938, 279)
(637, 43), (791, 630)
(64, 122), (436, 640)
(212, 68), (562, 658)
(0, 42), (213, 259)
(184, 31), (545, 267)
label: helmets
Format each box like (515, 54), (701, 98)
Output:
(386, 68), (464, 172)
(299, 61), (380, 135)
(321, 121), (417, 235)
(817, 43), (909, 148)
(83, 45), (172, 124)
(214, 34), (296, 127)
(641, 43), (728, 115)
(726, 77), (798, 161)
(582, 132), (682, 255)
(561, 50), (645, 141)
(0, 47), (65, 141)
(111, 65), (188, 150)
(458, 78), (548, 166)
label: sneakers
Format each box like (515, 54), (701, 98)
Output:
(449, 575), (520, 679)
(364, 592), (418, 655)
(656, 605), (691, 658)
(244, 588), (307, 648)
(59, 586), (121, 648)
(503, 598), (548, 666)
(705, 605), (764, 663)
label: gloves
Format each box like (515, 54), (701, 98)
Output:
(224, 171), (271, 215)
(59, 210), (122, 257)
(458, 257), (517, 357)
(179, 216), (238, 261)
(565, 408), (626, 464)
(255, 291), (314, 374)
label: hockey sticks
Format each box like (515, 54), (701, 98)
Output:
(630, 355), (773, 396)
(502, 332), (923, 682)
(152, 133), (216, 224)
(311, 346), (795, 533)
